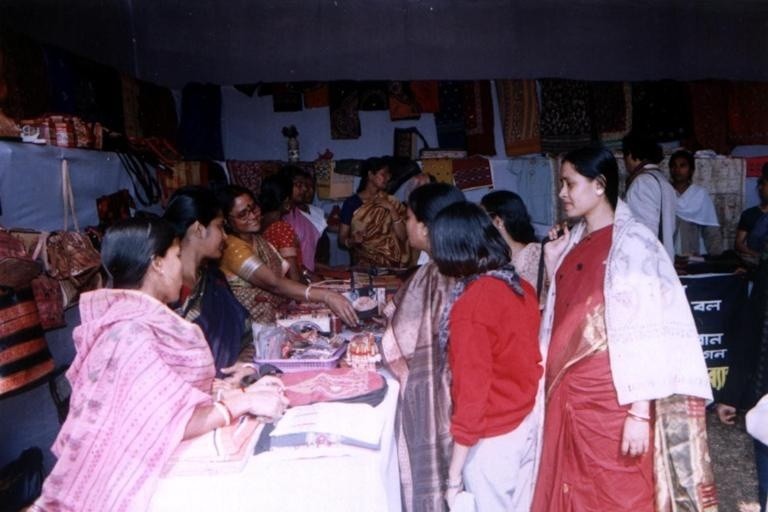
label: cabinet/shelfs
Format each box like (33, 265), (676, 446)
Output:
(144, 266), (402, 512)
(676, 255), (750, 409)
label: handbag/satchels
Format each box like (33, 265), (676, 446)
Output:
(61, 159), (100, 275)
(0, 230), (41, 289)
(33, 229), (63, 279)
(0, 447), (46, 512)
(96, 190), (135, 228)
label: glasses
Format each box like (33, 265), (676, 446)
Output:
(295, 181), (304, 188)
(229, 202), (259, 220)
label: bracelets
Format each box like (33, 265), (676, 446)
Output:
(344, 239), (353, 248)
(305, 286), (311, 301)
(627, 410), (652, 422)
(217, 389), (224, 401)
(445, 478), (463, 489)
(392, 216), (404, 225)
(212, 400), (233, 428)
(241, 387), (244, 393)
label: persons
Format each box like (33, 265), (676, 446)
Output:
(23, 216), (290, 512)
(278, 164), (321, 273)
(218, 185), (360, 329)
(426, 200), (545, 512)
(619, 132), (678, 267)
(665, 150), (723, 259)
(161, 185), (261, 387)
(530, 141), (714, 512)
(478, 189), (549, 311)
(717, 260), (768, 511)
(733, 173), (768, 266)
(337, 156), (410, 269)
(379, 180), (466, 512)
(299, 173), (329, 235)
(256, 174), (311, 285)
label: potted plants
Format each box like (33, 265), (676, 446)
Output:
(282, 124), (300, 163)
